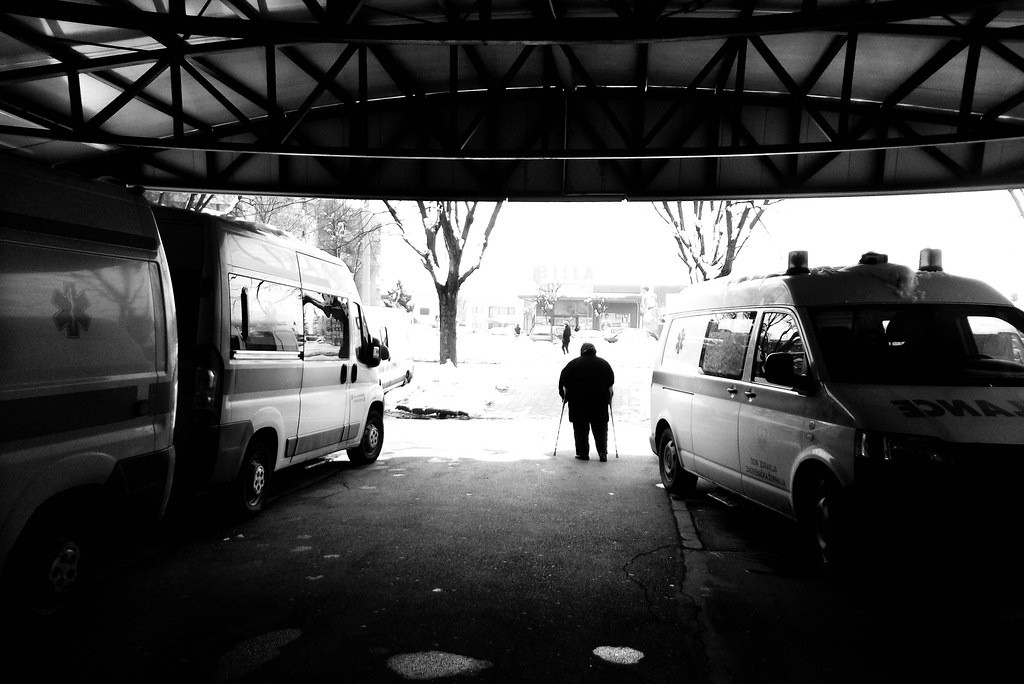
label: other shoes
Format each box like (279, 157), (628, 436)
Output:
(599, 453), (607, 462)
(575, 454), (589, 460)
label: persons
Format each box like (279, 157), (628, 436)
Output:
(562, 321), (571, 354)
(574, 326), (579, 331)
(559, 343), (614, 462)
(514, 324), (521, 334)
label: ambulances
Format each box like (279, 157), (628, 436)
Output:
(644, 248), (1024, 583)
(149, 204), (385, 522)
(1, 151), (179, 657)
